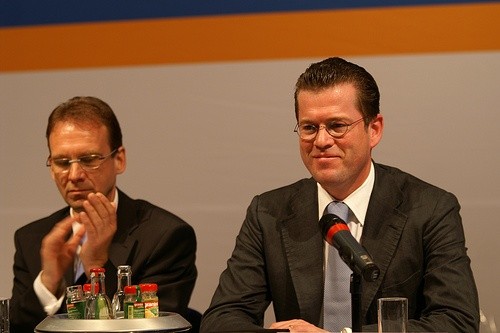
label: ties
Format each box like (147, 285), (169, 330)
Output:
(323, 201), (352, 333)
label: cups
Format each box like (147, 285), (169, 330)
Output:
(0, 298), (10, 333)
(377, 297), (408, 333)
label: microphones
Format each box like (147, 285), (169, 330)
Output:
(318, 213), (380, 282)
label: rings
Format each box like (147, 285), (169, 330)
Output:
(289, 325), (294, 328)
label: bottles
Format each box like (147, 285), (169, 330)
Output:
(85, 268), (114, 319)
(124, 284), (160, 320)
(66, 284), (100, 319)
(110, 265), (131, 318)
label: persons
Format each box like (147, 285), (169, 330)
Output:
(9, 96), (198, 333)
(200, 57), (480, 333)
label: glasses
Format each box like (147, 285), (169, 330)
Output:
(46, 148), (118, 170)
(294, 114), (371, 141)
